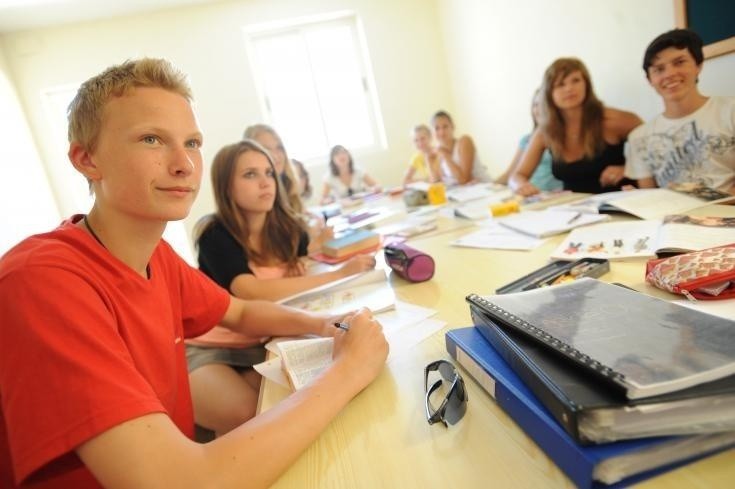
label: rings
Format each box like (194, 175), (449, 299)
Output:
(608, 175), (615, 179)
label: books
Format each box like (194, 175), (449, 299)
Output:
(446, 278), (734, 489)
(252, 182), (734, 391)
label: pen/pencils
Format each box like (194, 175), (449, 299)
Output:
(325, 215), (327, 226)
(334, 321), (350, 330)
(373, 237), (385, 257)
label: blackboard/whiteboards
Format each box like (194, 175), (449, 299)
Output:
(673, 0), (735, 61)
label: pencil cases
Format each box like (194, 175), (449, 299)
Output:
(494, 256), (610, 295)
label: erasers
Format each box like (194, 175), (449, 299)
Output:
(571, 261), (600, 276)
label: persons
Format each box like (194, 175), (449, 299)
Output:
(493, 88), (564, 193)
(243, 124), (313, 199)
(321, 145), (384, 205)
(621, 29), (735, 198)
(401, 125), (439, 183)
(1, 57), (390, 489)
(423, 112), (490, 185)
(290, 158), (319, 206)
(190, 140), (377, 303)
(508, 58), (645, 197)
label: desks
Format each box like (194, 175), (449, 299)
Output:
(255, 184), (735, 489)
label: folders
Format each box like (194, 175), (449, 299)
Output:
(468, 307), (734, 446)
(444, 328), (735, 489)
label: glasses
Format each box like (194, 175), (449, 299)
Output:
(424, 359), (468, 426)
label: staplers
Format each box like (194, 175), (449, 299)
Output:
(656, 246), (693, 258)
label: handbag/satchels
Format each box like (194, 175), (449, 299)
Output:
(646, 243), (734, 302)
